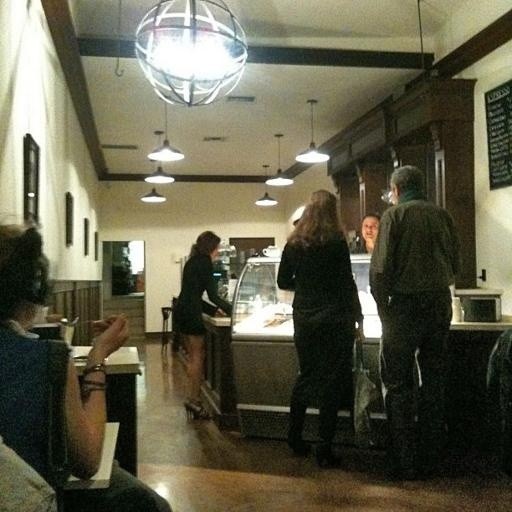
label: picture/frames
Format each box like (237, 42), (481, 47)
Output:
(24, 132), (41, 223)
(66, 191), (100, 261)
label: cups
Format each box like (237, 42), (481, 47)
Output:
(451, 296), (461, 323)
(64, 324), (74, 346)
(262, 246), (280, 259)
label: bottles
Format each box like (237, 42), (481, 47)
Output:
(228, 274), (238, 301)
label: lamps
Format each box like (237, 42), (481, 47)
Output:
(254, 165), (278, 204)
(147, 95), (184, 162)
(138, 186), (168, 204)
(141, 163), (180, 186)
(135, 1), (247, 111)
(265, 133), (294, 186)
(294, 99), (333, 164)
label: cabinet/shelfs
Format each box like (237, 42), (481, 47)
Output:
(230, 256), (390, 449)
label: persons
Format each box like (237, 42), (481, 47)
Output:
(277, 188), (366, 471)
(171, 230), (235, 422)
(0, 221), (132, 512)
(350, 213), (383, 252)
(367, 163), (466, 483)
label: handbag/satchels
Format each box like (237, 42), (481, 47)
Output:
(354, 335), (380, 433)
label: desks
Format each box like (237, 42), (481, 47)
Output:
(62, 346), (142, 476)
(59, 420), (120, 494)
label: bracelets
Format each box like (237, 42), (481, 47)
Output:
(81, 379), (110, 391)
(83, 363), (106, 373)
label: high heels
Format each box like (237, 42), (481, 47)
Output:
(185, 398), (211, 420)
(316, 439), (335, 467)
